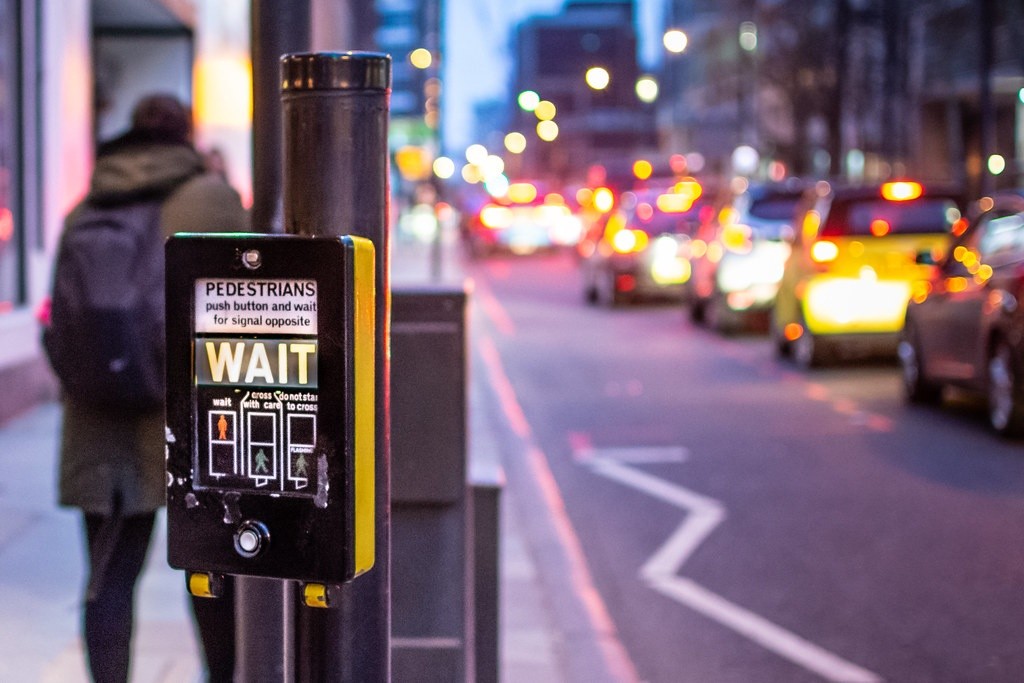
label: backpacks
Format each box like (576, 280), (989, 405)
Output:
(41, 188), (167, 414)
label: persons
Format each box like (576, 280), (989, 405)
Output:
(41, 93), (248, 683)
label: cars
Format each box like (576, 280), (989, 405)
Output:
(569, 173), (1023, 440)
